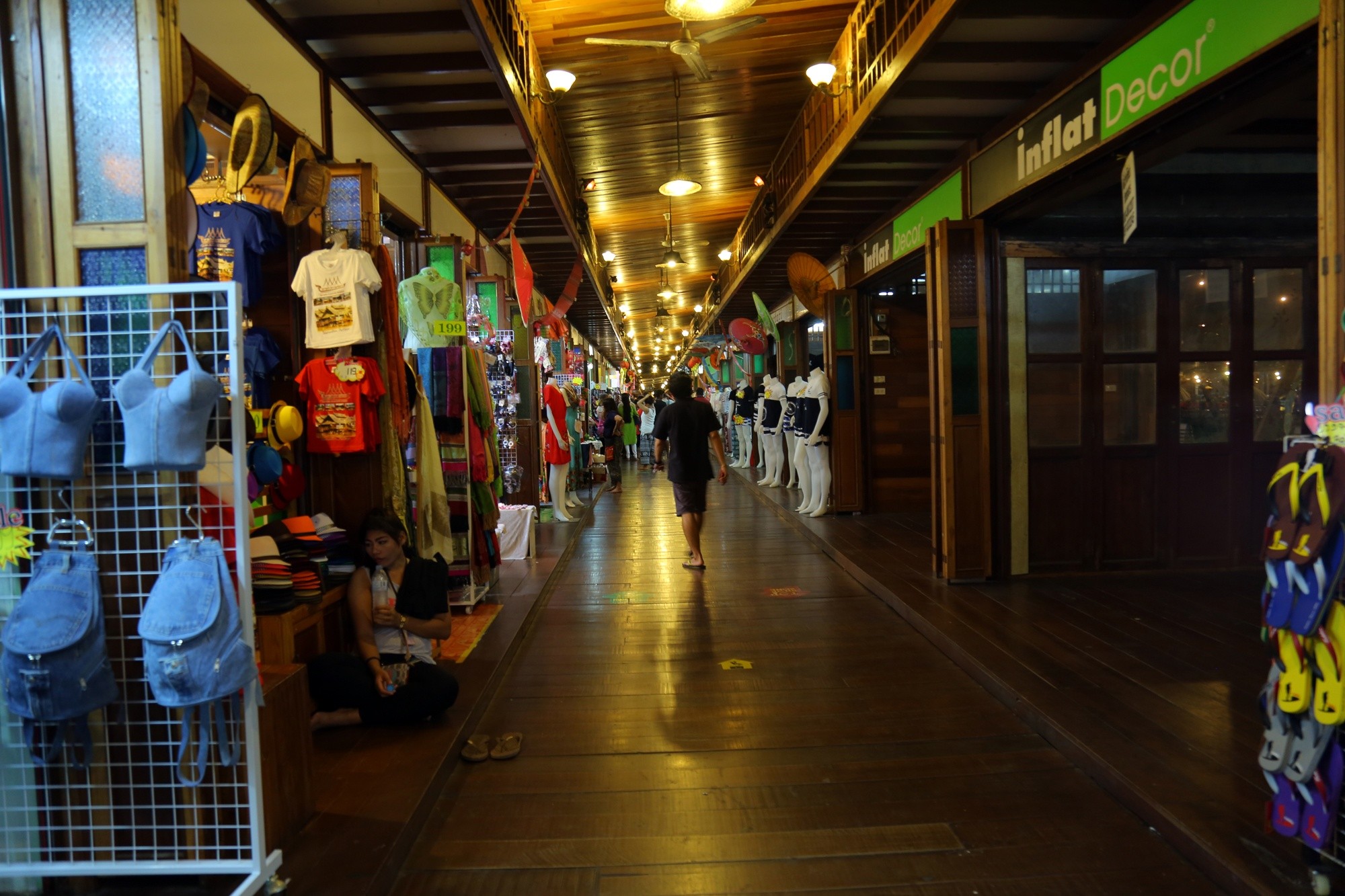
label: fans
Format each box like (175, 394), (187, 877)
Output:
(659, 213), (709, 247)
(584, 15), (766, 83)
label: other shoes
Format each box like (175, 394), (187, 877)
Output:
(633, 458), (639, 460)
(624, 458), (630, 461)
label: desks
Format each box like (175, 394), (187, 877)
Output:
(498, 505), (536, 558)
(257, 581), (349, 663)
(582, 439), (602, 492)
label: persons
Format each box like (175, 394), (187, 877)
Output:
(543, 367), (831, 522)
(397, 267), (462, 354)
(595, 371), (728, 571)
(305, 508), (460, 730)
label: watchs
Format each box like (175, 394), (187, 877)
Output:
(399, 614), (406, 628)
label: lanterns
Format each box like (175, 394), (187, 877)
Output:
(619, 356), (701, 383)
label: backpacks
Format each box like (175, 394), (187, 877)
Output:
(138, 537), (259, 786)
(0, 540), (120, 776)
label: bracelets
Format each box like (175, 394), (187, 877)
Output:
(366, 656), (382, 666)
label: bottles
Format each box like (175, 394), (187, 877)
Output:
(372, 565), (389, 610)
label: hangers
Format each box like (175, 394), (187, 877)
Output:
(206, 175), (234, 205)
(325, 346), (356, 366)
(318, 232), (347, 261)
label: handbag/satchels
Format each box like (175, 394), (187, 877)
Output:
(633, 414), (641, 426)
(381, 664), (409, 685)
(605, 446), (613, 461)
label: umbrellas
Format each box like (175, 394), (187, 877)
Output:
(685, 313), (726, 369)
(787, 252), (848, 319)
(510, 230), (543, 329)
(752, 292), (793, 341)
(729, 318), (768, 355)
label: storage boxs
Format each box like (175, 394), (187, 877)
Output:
(591, 454), (608, 481)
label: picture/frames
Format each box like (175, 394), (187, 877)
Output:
(532, 287), (546, 317)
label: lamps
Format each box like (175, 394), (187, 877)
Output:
(597, 196), (689, 374)
(664, 0), (756, 21)
(576, 178), (597, 218)
(753, 173), (772, 192)
(657, 71), (702, 196)
(710, 273), (717, 280)
(805, 63), (855, 98)
(533, 70), (576, 106)
(693, 304), (706, 317)
(717, 248), (741, 267)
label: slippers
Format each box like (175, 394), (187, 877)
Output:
(683, 559), (706, 569)
(489, 731), (522, 757)
(1258, 443), (1345, 849)
(462, 735), (489, 759)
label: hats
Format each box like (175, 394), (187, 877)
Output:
(247, 401), (347, 616)
